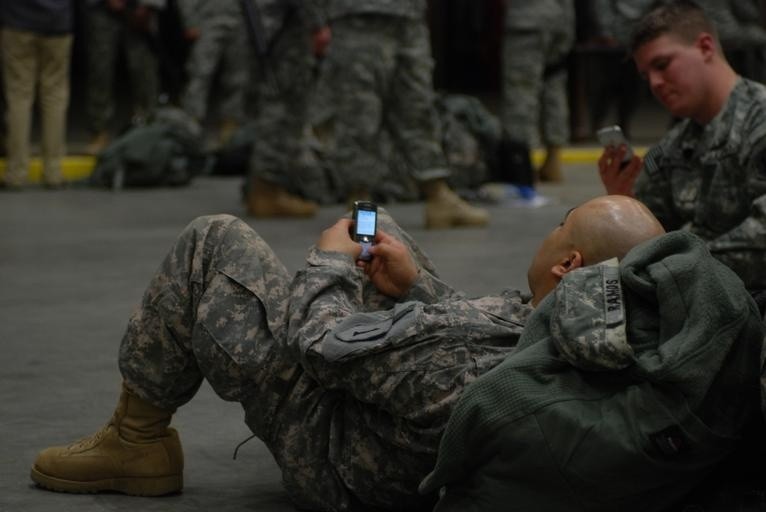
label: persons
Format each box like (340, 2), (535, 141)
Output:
(26, 193), (670, 512)
(2, 0), (766, 231)
(594, 0), (766, 314)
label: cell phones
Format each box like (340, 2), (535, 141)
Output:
(350, 200), (377, 262)
(597, 126), (634, 170)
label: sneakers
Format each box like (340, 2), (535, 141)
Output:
(0, 167), (69, 189)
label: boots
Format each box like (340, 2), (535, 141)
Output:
(29, 384), (186, 497)
(244, 180), (321, 218)
(421, 182), (491, 234)
